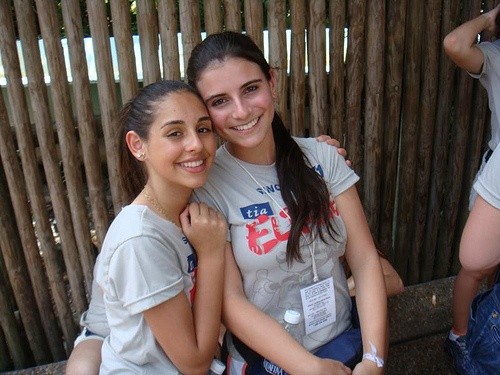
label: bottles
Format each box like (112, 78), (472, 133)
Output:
(264, 310), (303, 375)
(210, 359), (226, 375)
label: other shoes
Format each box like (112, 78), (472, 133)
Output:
(443, 331), (481, 375)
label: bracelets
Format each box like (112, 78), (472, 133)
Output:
(363, 343), (385, 367)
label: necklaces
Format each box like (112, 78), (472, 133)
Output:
(143, 187), (181, 228)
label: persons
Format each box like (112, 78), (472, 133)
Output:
(188, 32), (388, 375)
(95, 80), (351, 375)
(443, 4), (500, 357)
(458, 143), (500, 375)
